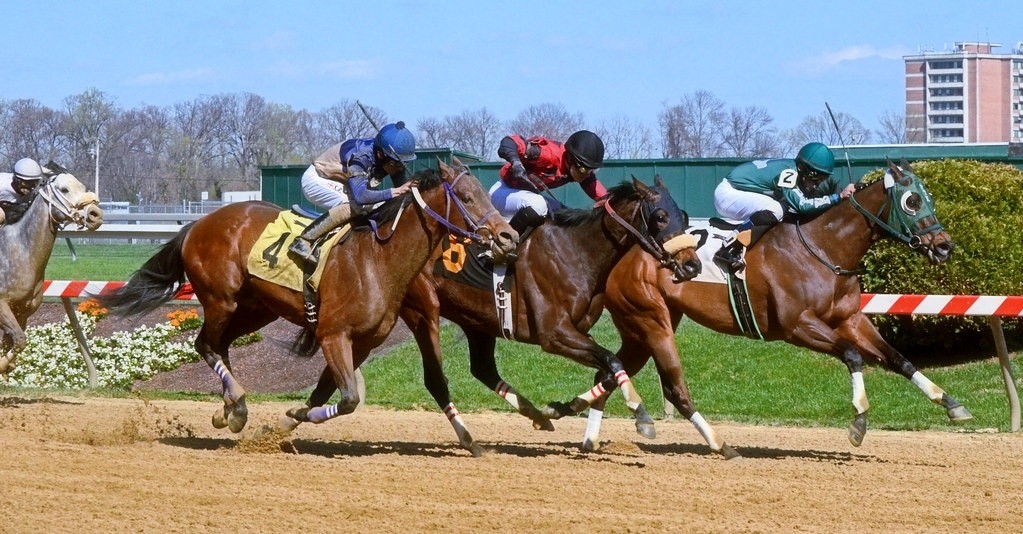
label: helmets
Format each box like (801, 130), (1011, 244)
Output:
(798, 142), (835, 175)
(373, 121), (418, 162)
(14, 158), (43, 180)
(564, 130), (604, 169)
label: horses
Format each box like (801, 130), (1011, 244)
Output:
(94, 155), (519, 460)
(397, 172), (705, 460)
(577, 155), (975, 464)
(0, 162), (101, 376)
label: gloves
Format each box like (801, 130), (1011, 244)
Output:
(511, 159), (525, 179)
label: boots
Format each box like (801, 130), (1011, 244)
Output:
(288, 212), (337, 263)
(506, 207), (531, 263)
(713, 209), (777, 267)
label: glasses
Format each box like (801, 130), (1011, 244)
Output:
(387, 156), (406, 168)
(574, 157), (593, 175)
(808, 169), (830, 182)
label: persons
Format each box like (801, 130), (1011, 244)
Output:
(486, 130), (610, 260)
(0, 158), (43, 226)
(714, 143), (856, 266)
(288, 121), (418, 262)
